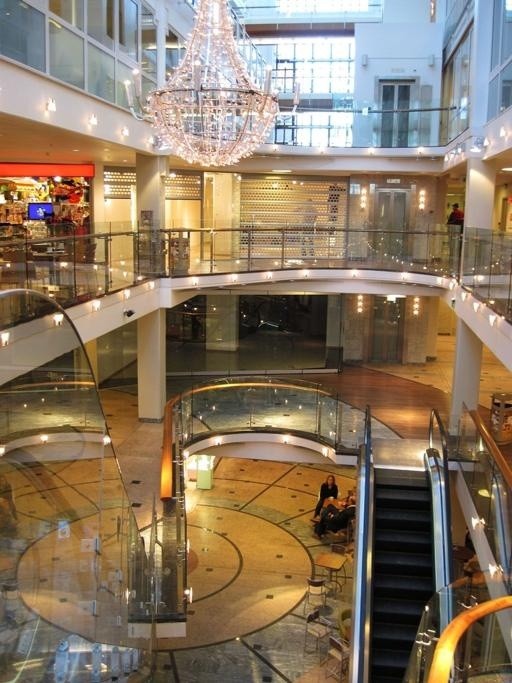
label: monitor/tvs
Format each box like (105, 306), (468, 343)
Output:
(28, 203), (53, 220)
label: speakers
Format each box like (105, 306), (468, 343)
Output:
(428, 55), (434, 65)
(362, 55), (368, 66)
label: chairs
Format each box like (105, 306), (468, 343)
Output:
(321, 543), (347, 585)
(303, 577), (326, 615)
(325, 636), (350, 682)
(303, 609), (332, 667)
(328, 526), (350, 543)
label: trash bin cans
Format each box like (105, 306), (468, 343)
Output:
(490, 393), (511, 446)
(168, 238), (190, 276)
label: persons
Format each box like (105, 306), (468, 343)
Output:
(447, 203), (464, 255)
(299, 198), (317, 255)
(314, 475), (338, 518)
(310, 495), (355, 539)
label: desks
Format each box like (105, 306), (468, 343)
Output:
(314, 551), (347, 600)
(322, 498), (348, 512)
